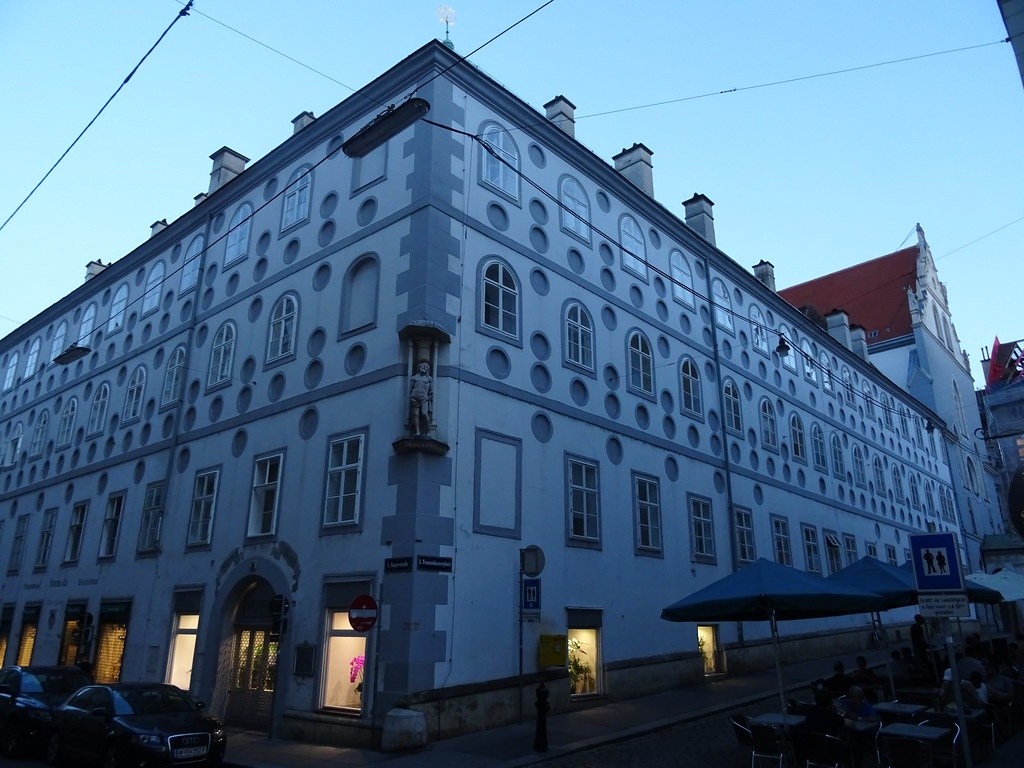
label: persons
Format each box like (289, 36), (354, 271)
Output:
(788, 614), (1024, 768)
(408, 362), (434, 436)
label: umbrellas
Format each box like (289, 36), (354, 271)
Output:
(660, 557), (1024, 622)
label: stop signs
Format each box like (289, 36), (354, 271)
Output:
(348, 594), (379, 633)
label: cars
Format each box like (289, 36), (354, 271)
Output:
(47, 682), (228, 768)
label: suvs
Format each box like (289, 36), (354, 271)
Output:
(0, 664), (95, 762)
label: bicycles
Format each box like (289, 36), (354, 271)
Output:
(867, 619), (889, 649)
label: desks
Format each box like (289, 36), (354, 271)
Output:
(748, 712), (806, 731)
(924, 708), (987, 722)
(874, 703), (926, 723)
(879, 723), (952, 768)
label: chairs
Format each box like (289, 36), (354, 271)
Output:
(730, 698), (1016, 768)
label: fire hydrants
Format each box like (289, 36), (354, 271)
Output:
(532, 680), (550, 754)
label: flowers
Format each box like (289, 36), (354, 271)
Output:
(568, 638), (595, 688)
(698, 632), (709, 664)
(349, 656), (365, 694)
